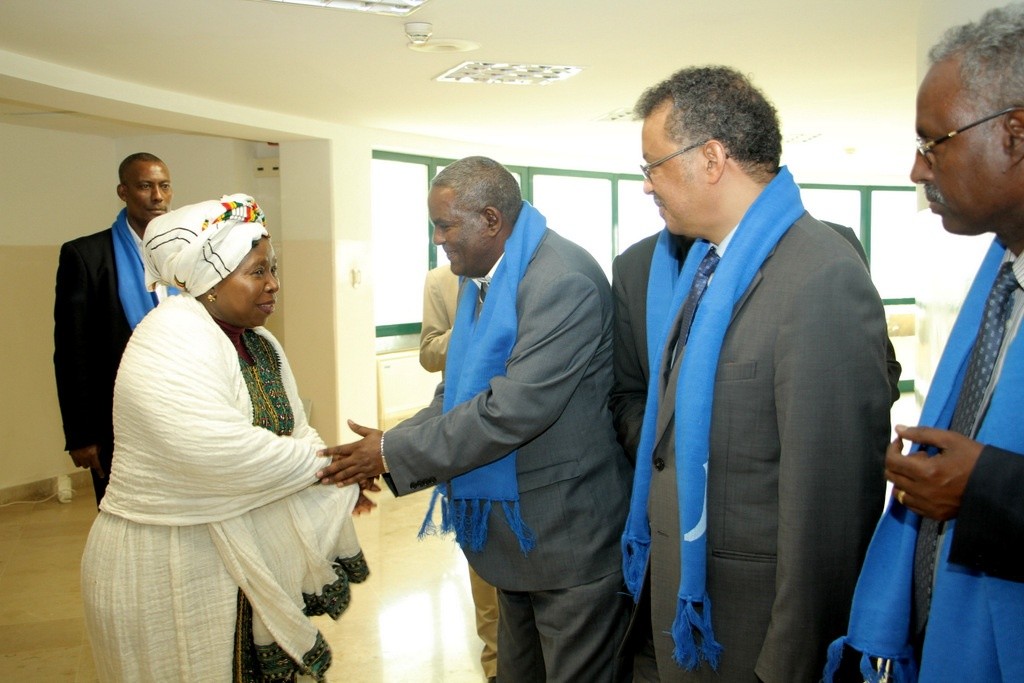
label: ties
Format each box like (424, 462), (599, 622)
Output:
(481, 282), (489, 299)
(912, 262), (1019, 635)
(672, 247), (721, 375)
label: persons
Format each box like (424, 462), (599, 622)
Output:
(611, 220), (902, 468)
(886, 0), (1024, 683)
(316, 155), (637, 683)
(79, 194), (381, 683)
(421, 264), (498, 683)
(631, 65), (892, 683)
(53, 152), (180, 513)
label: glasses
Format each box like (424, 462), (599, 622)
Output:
(918, 107), (1020, 167)
(641, 141), (706, 184)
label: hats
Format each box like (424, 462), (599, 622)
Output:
(143, 194), (271, 295)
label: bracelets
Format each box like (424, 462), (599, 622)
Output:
(381, 431), (390, 473)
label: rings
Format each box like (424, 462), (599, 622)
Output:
(898, 490), (906, 504)
